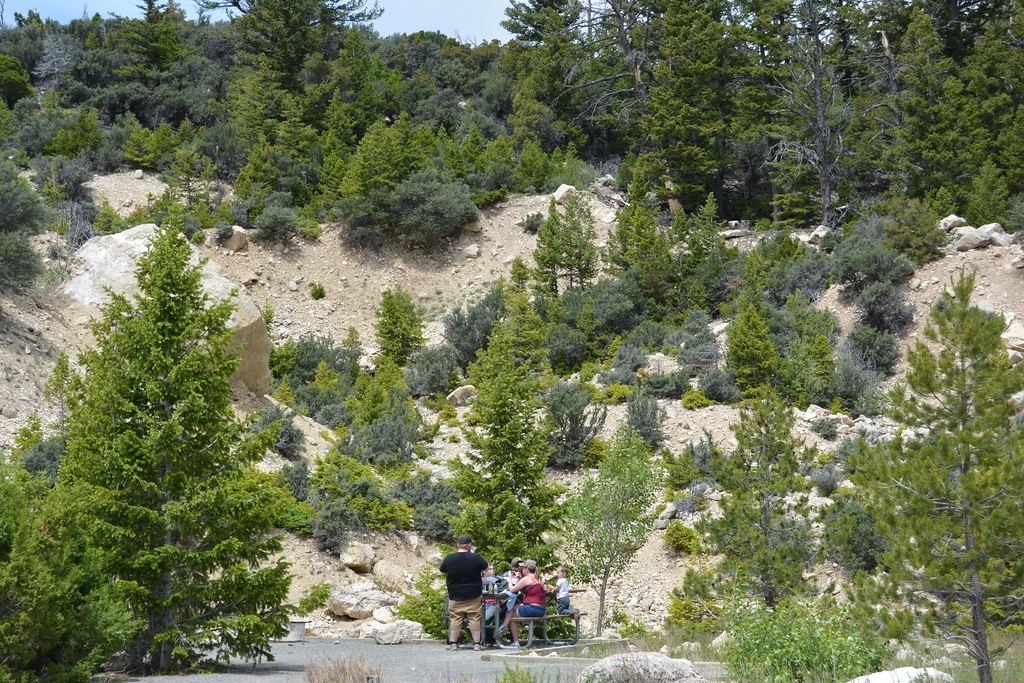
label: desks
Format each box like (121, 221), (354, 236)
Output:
(457, 589), (588, 649)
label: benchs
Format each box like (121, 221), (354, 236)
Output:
(511, 612), (589, 623)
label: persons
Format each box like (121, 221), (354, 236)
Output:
(492, 558), (546, 649)
(549, 566), (579, 621)
(439, 534), (490, 651)
(469, 560), (498, 649)
(503, 556), (523, 645)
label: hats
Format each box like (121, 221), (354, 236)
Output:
(458, 534), (475, 544)
(512, 557), (522, 564)
(519, 559), (536, 569)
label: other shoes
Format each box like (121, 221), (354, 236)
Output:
(493, 626), (509, 640)
(446, 643), (457, 651)
(573, 610), (580, 620)
(474, 644), (482, 651)
(508, 642), (520, 647)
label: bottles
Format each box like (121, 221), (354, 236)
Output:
(488, 581), (498, 594)
